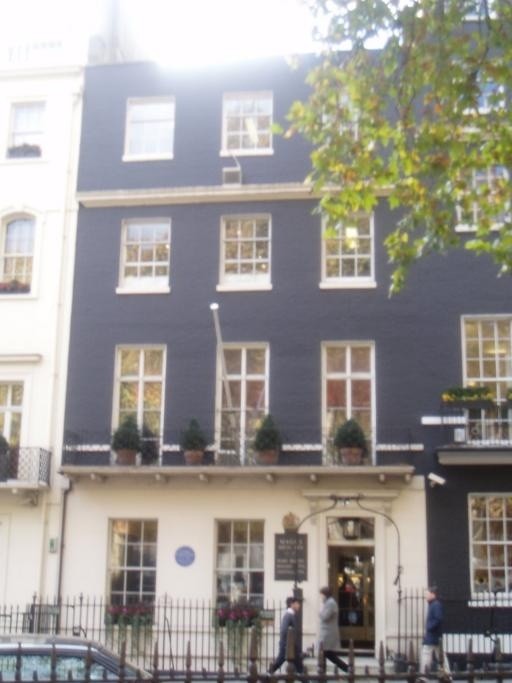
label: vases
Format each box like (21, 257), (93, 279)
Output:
(441, 400), (496, 408)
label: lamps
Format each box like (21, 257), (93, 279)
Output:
(342, 518), (362, 540)
(427, 472), (446, 487)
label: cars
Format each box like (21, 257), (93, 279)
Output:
(0, 633), (153, 682)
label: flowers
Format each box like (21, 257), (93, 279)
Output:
(106, 601), (154, 647)
(441, 385), (498, 402)
(214, 600), (263, 636)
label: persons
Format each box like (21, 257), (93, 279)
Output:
(315, 585), (349, 673)
(414, 583), (454, 682)
(263, 594), (306, 676)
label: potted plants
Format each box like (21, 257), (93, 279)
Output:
(251, 414), (282, 464)
(334, 418), (366, 465)
(180, 416), (208, 465)
(112, 411), (143, 466)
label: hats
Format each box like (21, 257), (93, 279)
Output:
(427, 587), (439, 596)
(285, 596), (303, 606)
(319, 586), (333, 597)
